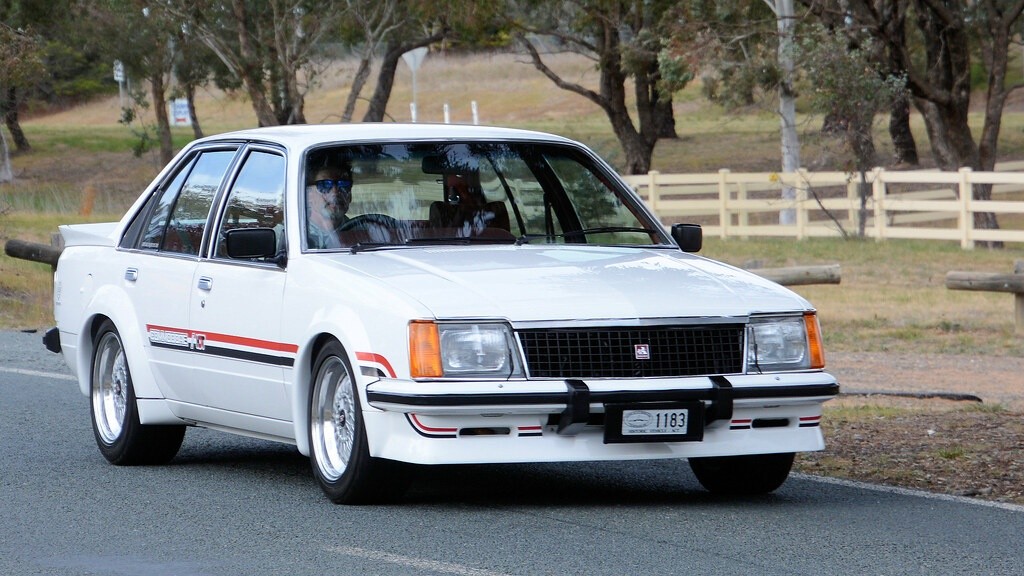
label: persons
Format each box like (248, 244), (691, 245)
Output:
(255, 156), (372, 270)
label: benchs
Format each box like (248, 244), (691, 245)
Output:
(156, 222), (276, 263)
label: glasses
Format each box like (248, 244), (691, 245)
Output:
(307, 178), (354, 195)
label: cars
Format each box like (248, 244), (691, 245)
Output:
(37, 120), (842, 505)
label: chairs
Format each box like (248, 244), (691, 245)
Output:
(430, 201), (510, 234)
